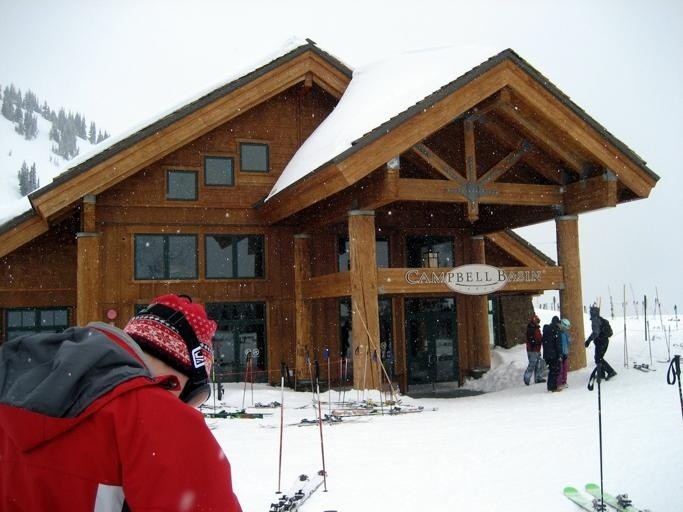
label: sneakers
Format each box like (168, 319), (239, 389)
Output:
(547, 384), (569, 392)
(524, 376), (529, 385)
(597, 371), (617, 381)
(535, 379), (546, 383)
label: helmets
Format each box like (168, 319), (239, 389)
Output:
(561, 319), (570, 328)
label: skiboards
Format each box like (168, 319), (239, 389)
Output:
(563, 484), (649, 512)
(199, 400), (438, 426)
(269, 471), (328, 511)
(657, 358), (683, 364)
(632, 364), (656, 372)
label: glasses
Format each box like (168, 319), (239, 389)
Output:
(179, 376), (211, 406)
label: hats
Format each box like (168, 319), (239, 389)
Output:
(123, 293), (217, 374)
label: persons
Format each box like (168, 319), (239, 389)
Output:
(0, 295), (242, 512)
(523, 316), (546, 386)
(556, 318), (571, 390)
(585, 307), (617, 381)
(543, 316), (562, 392)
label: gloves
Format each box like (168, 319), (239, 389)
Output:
(585, 338), (591, 347)
